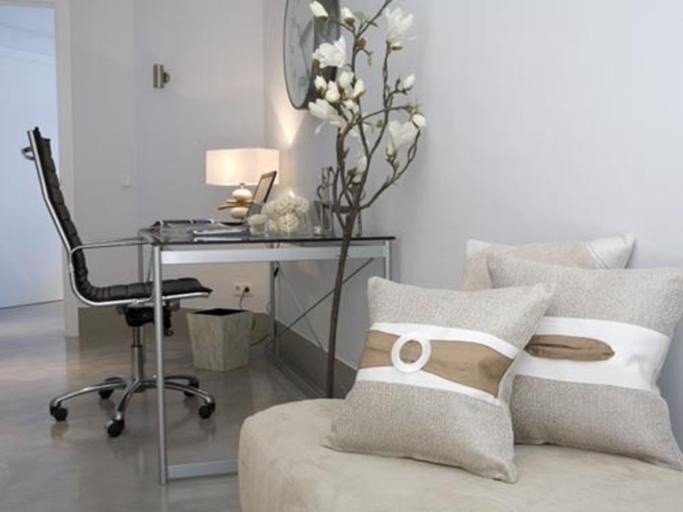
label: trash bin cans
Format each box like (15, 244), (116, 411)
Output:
(186, 308), (253, 372)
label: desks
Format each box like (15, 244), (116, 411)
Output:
(137, 227), (398, 487)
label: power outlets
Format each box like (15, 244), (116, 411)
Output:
(232, 281), (253, 297)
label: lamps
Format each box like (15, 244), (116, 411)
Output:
(203, 146), (281, 220)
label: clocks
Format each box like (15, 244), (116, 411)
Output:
(281, 0), (340, 110)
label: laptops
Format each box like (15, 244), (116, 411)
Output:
(191, 170), (277, 235)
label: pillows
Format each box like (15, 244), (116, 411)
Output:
(484, 249), (681, 472)
(317, 275), (559, 487)
(455, 234), (639, 293)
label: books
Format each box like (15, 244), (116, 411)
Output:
(150, 218), (217, 227)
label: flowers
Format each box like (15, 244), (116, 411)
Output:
(298, 0), (427, 400)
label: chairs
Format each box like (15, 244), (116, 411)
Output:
(18, 124), (218, 439)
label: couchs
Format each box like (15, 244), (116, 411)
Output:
(235, 396), (681, 512)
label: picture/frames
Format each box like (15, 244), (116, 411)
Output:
(244, 170), (278, 219)
